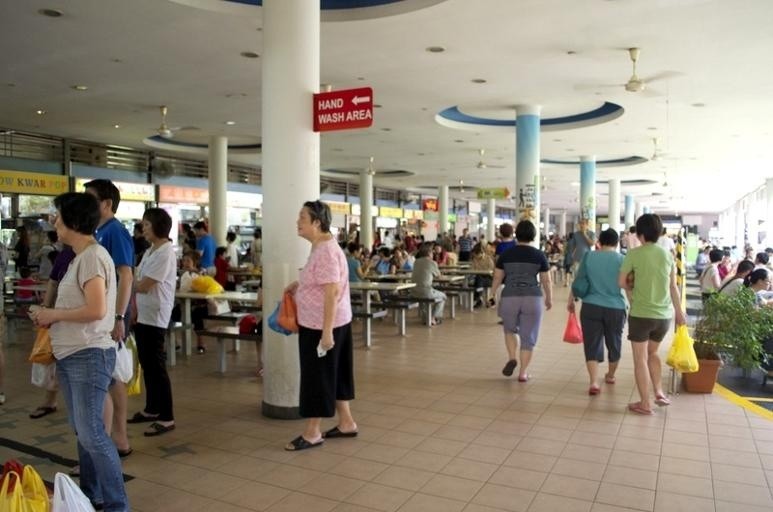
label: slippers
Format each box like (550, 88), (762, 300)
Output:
(424, 318), (442, 325)
(502, 359), (531, 381)
(285, 436), (325, 451)
(322, 425), (358, 438)
(126, 411), (160, 423)
(143, 423), (175, 437)
(655, 398), (672, 407)
(605, 372), (616, 383)
(630, 401), (654, 415)
(589, 386), (601, 395)
(29, 406), (56, 418)
(70, 447), (133, 476)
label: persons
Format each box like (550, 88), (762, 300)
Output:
(486, 220), (553, 382)
(1, 226), (58, 279)
(618, 213), (686, 415)
(25, 193), (127, 512)
(537, 225), (676, 287)
(84, 178), (134, 476)
(336, 222), (517, 326)
(178, 221), (263, 353)
(685, 225), (773, 308)
(30, 244), (76, 417)
(283, 200), (358, 451)
(126, 205), (176, 437)
(567, 228), (625, 395)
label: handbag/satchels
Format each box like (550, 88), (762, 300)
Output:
(207, 300), (231, 315)
(190, 275), (225, 294)
(46, 249), (59, 263)
(123, 333), (141, 396)
(240, 315), (257, 333)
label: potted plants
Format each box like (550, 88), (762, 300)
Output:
(682, 284), (773, 394)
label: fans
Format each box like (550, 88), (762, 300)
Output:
(577, 45), (682, 98)
(139, 103), (201, 137)
(150, 159), (176, 182)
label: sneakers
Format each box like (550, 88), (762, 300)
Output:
(176, 345), (180, 352)
(197, 343), (205, 354)
(474, 299), (482, 308)
(0, 393), (5, 405)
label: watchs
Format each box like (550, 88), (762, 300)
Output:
(116, 314), (125, 320)
